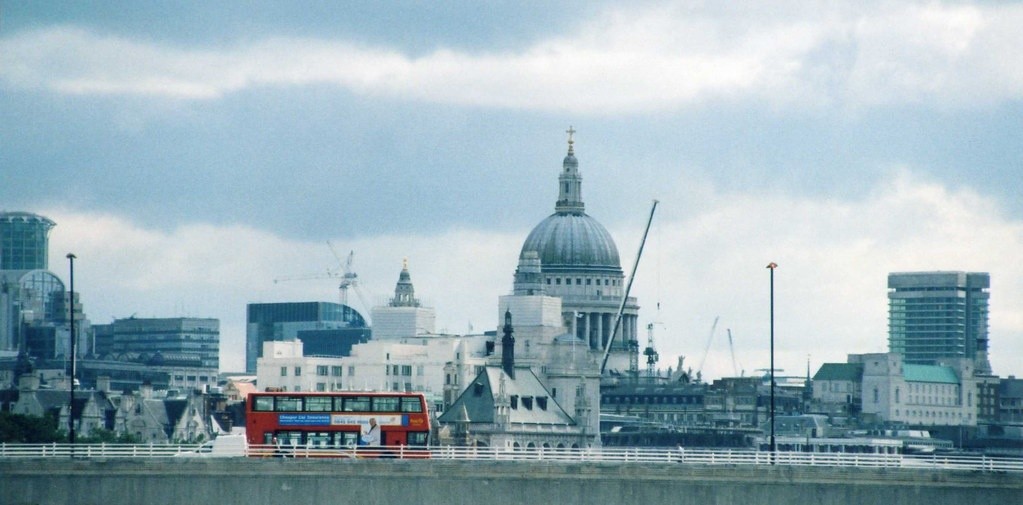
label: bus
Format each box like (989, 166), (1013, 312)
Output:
(246, 389), (432, 459)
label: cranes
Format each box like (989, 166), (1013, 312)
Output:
(272, 238), (373, 319)
(600, 198), (662, 389)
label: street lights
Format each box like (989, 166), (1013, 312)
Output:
(65, 252), (78, 456)
(766, 263), (778, 466)
